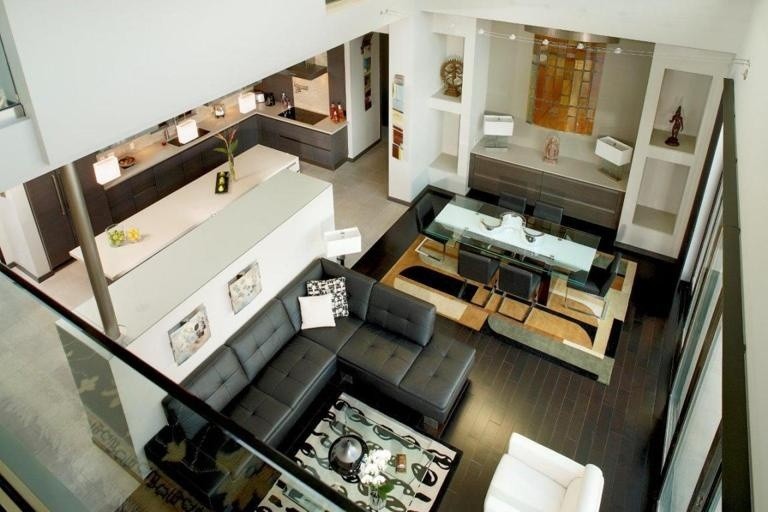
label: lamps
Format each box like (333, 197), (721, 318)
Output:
(324, 225), (366, 261)
(481, 111), (516, 151)
(595, 133), (631, 183)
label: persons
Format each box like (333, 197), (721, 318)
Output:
(667, 104), (684, 141)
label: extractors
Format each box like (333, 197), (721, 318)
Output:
(277, 62), (327, 80)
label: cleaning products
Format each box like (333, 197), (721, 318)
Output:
(330, 102), (344, 123)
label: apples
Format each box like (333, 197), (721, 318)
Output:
(108, 231), (125, 247)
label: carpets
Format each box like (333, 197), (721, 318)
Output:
(378, 233), (639, 387)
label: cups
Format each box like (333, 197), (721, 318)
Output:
(123, 221), (141, 244)
(106, 223), (125, 247)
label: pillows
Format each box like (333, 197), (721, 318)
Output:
(296, 276), (349, 331)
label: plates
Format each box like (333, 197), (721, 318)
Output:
(215, 170), (230, 194)
(118, 155), (136, 170)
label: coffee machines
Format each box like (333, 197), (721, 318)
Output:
(263, 92), (275, 106)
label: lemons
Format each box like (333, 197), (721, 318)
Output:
(127, 226), (139, 241)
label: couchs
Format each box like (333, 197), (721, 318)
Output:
(143, 257), (477, 512)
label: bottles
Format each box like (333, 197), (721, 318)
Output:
(286, 97), (292, 108)
(280, 91), (287, 108)
(330, 101), (345, 124)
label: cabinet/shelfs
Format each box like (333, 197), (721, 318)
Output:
(258, 117), (348, 171)
(467, 153), (623, 229)
(91, 116), (258, 226)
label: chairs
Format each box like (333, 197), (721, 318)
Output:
(416, 181), (624, 308)
(481, 429), (605, 512)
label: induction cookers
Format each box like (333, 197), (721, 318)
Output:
(278, 106), (328, 126)
